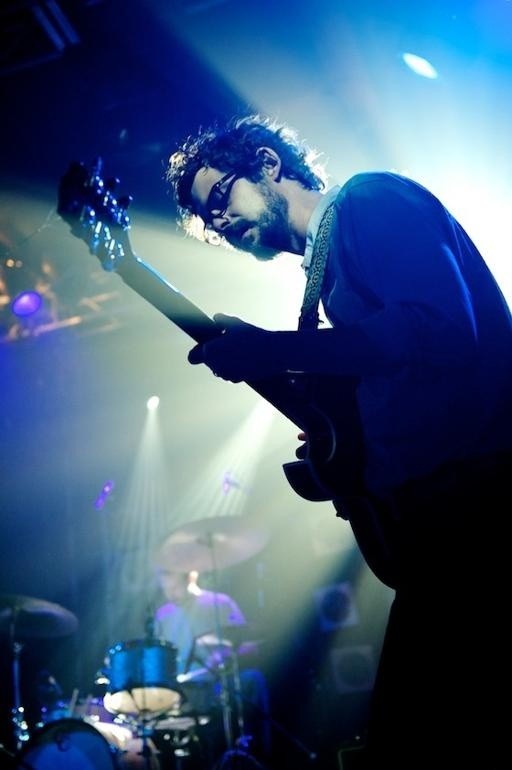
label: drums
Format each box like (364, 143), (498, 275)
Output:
(41, 698), (112, 720)
(145, 682), (213, 732)
(102, 638), (182, 716)
(18, 718), (159, 768)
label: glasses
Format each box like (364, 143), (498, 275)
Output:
(204, 171), (236, 248)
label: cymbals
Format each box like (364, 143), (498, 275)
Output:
(160, 516), (268, 572)
(194, 627), (269, 645)
(1, 594), (79, 639)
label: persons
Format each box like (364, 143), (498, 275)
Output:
(165, 113), (512, 765)
(155, 567), (248, 669)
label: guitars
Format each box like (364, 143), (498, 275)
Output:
(55, 155), (400, 589)
